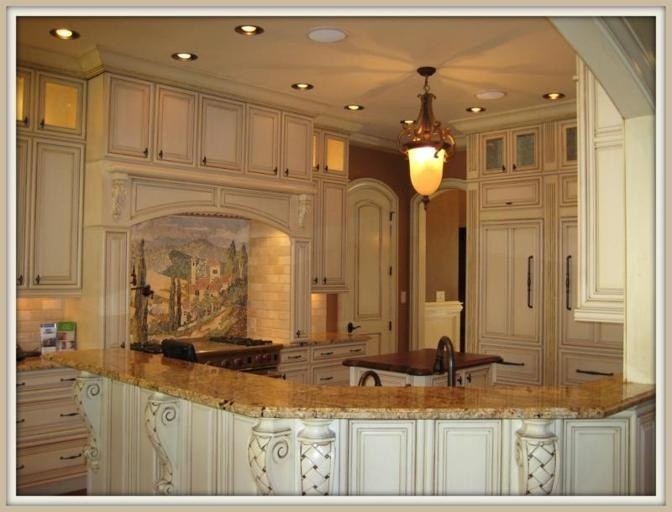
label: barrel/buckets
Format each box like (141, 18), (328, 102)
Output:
(432, 335), (456, 387)
(358, 371), (381, 386)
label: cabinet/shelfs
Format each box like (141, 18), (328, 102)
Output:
(464, 123), (544, 388)
(312, 341), (368, 387)
(197, 91), (246, 171)
(278, 348), (312, 386)
(310, 124), (355, 295)
(546, 118), (623, 386)
(16, 61), (84, 304)
(84, 67), (199, 169)
(247, 102), (314, 183)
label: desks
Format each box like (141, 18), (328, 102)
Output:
(341, 348), (502, 388)
